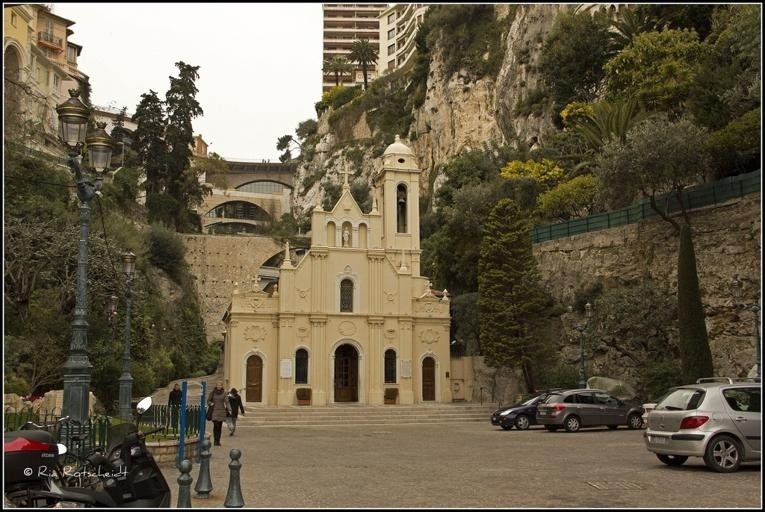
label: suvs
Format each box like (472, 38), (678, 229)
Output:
(644, 376), (761, 473)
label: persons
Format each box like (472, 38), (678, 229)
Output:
(208, 382), (234, 447)
(167, 383), (183, 431)
(227, 388), (247, 436)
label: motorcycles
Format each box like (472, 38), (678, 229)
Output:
(2, 393), (175, 508)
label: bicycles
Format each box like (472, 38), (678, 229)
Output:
(16, 416), (107, 489)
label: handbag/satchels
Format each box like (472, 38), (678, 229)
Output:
(206, 403), (214, 420)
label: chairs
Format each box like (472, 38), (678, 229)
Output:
(726, 397), (743, 411)
(747, 393), (760, 412)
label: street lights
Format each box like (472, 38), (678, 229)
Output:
(565, 303), (596, 388)
(50, 88), (105, 450)
(120, 246), (135, 420)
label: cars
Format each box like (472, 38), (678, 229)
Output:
(487, 389), (657, 431)
